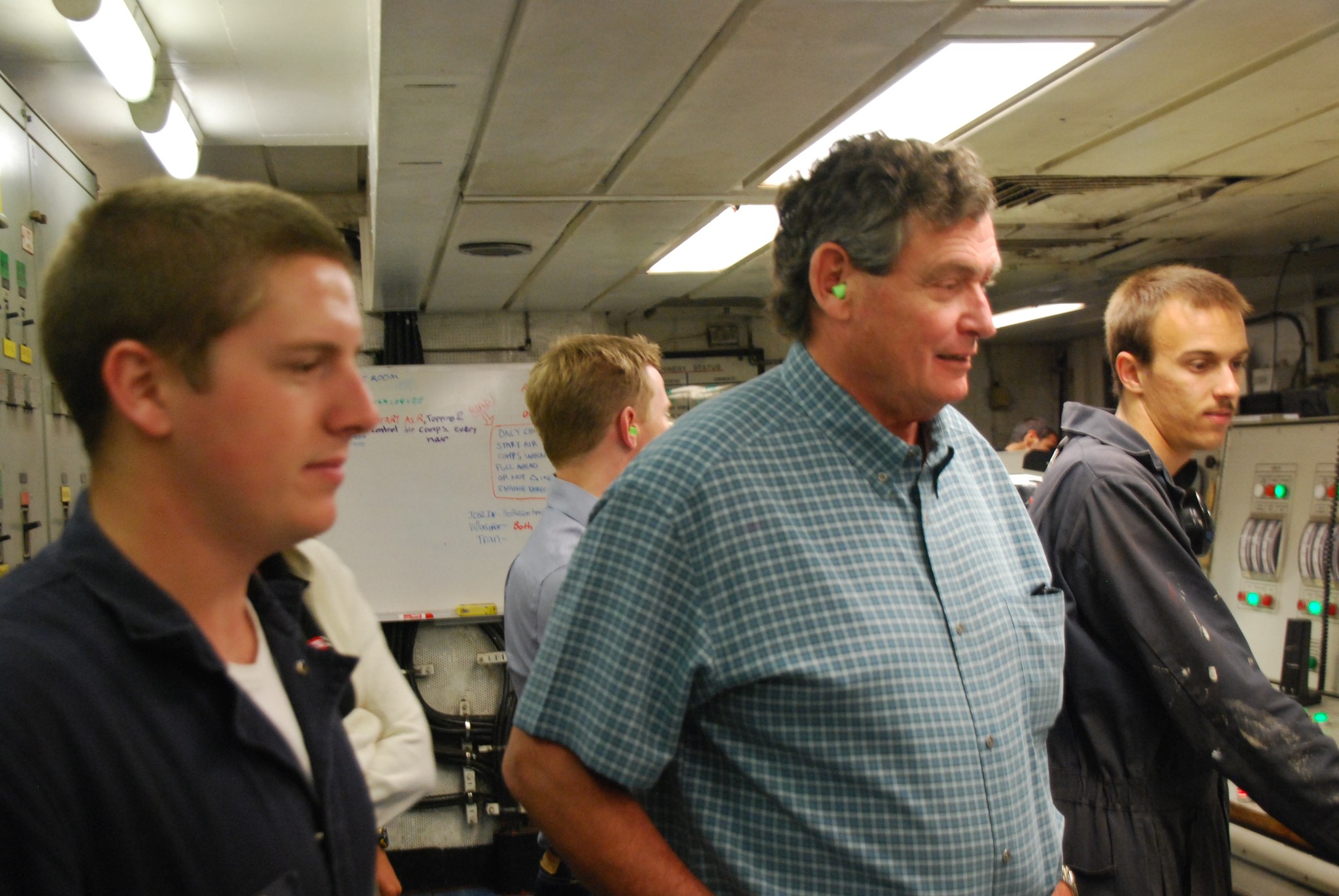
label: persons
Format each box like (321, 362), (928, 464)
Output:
(501, 129), (1081, 896)
(1, 177), (377, 896)
(1001, 419), (1058, 454)
(284, 538), (438, 896)
(1028, 264), (1339, 896)
(503, 334), (677, 894)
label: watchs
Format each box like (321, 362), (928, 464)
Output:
(376, 828), (390, 849)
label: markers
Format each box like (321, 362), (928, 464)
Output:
(398, 614), (433, 620)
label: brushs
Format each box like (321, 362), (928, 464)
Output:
(456, 602), (497, 615)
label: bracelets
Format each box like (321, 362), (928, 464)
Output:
(1061, 867), (1080, 896)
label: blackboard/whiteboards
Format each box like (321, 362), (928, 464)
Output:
(312, 364), (557, 621)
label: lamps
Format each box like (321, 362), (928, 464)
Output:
(128, 79), (204, 181)
(52, 1), (163, 105)
